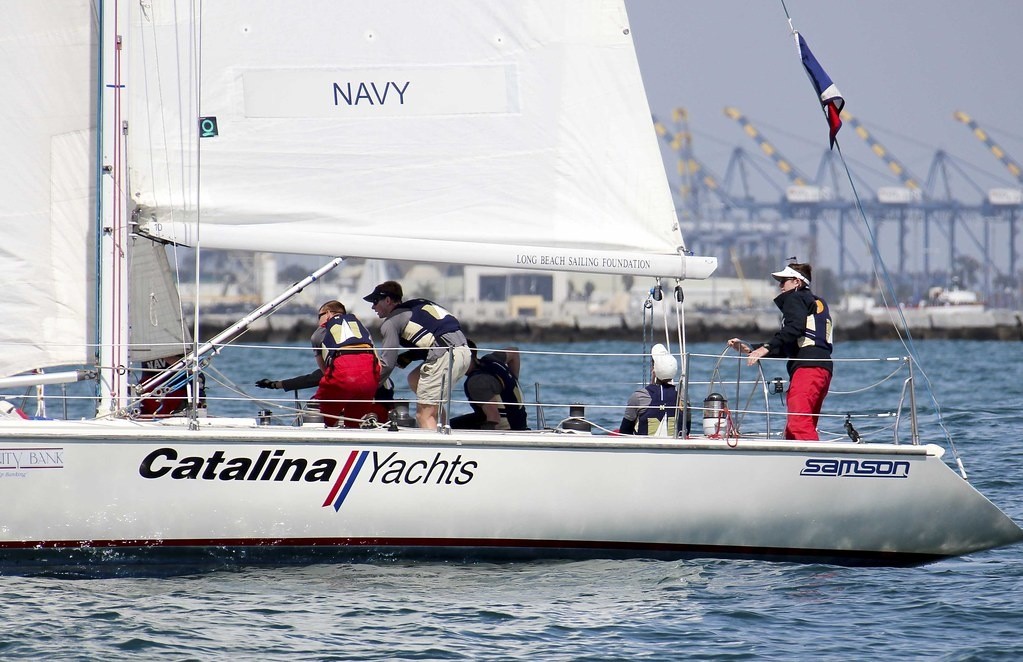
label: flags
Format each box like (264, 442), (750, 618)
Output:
(794, 29), (844, 150)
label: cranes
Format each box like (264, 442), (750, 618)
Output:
(650, 107), (1022, 311)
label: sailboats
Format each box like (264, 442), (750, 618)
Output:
(0, 1), (1022, 567)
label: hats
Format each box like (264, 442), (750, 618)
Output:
(362, 282), (403, 302)
(651, 344), (677, 381)
(770, 265), (810, 286)
(466, 337), (477, 352)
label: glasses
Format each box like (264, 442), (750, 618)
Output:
(373, 298), (387, 305)
(779, 277), (794, 284)
(318, 311), (336, 319)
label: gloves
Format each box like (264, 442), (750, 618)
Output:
(255, 379), (279, 390)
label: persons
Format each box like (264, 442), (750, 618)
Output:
(449, 338), (528, 430)
(618, 343), (691, 437)
(254, 368), (391, 424)
(310, 301), (381, 429)
(135, 355), (206, 418)
(363, 281), (471, 430)
(727, 264), (833, 441)
